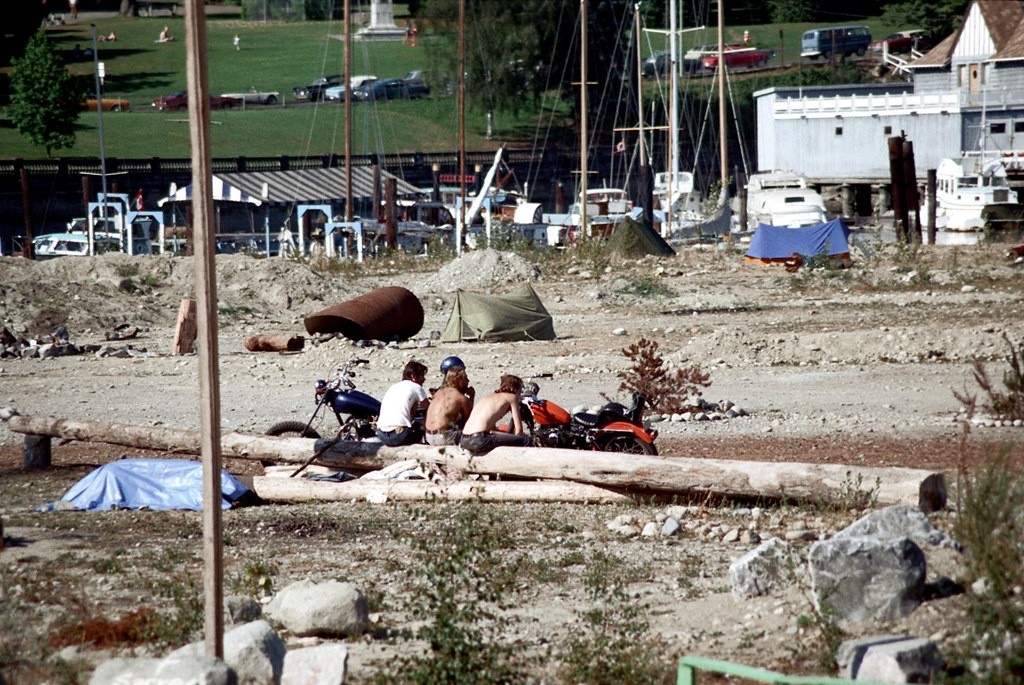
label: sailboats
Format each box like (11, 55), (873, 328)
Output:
(283, 1), (829, 261)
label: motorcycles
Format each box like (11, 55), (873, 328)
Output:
(259, 359), (439, 469)
(496, 374), (659, 457)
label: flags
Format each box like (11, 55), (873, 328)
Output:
(614, 140), (626, 154)
(136, 190), (143, 212)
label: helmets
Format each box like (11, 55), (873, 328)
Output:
(440, 356), (466, 374)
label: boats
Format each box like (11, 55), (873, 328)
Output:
(913, 83), (1024, 234)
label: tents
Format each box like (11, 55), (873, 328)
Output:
(603, 214), (677, 258)
(442, 280), (558, 344)
(743, 215), (852, 266)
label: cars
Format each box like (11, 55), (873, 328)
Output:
(56, 93), (130, 112)
(292, 74), (346, 103)
(324, 74), (379, 103)
(684, 44), (717, 61)
(150, 90), (239, 112)
(403, 69), (437, 83)
(701, 42), (770, 72)
(642, 50), (702, 78)
(353, 78), (431, 102)
(869, 29), (935, 56)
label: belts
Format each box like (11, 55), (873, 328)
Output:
(461, 430), (494, 438)
(425, 425), (449, 434)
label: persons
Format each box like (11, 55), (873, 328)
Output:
(159, 26), (174, 42)
(234, 33), (240, 50)
(460, 377), (531, 454)
(378, 361), (430, 447)
(97, 31), (116, 41)
(424, 365), (478, 446)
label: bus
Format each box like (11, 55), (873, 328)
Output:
(801, 25), (872, 61)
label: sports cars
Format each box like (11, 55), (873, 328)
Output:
(220, 86), (280, 106)
